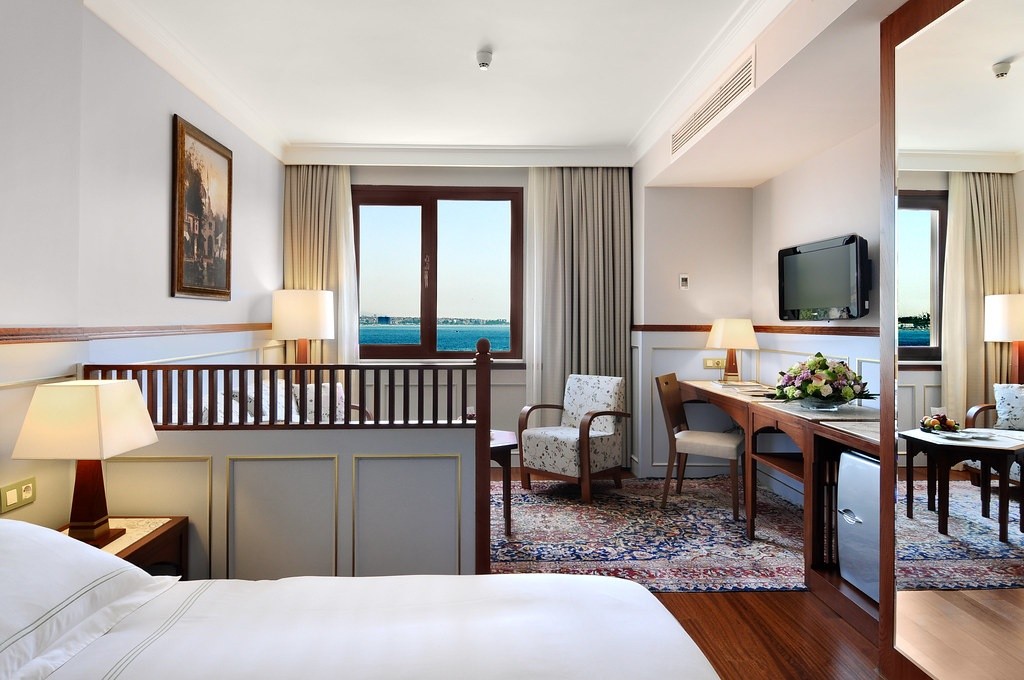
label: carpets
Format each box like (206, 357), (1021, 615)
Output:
(896, 480), (1024, 591)
(490, 473), (809, 593)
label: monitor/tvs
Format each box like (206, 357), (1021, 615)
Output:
(777, 235), (870, 321)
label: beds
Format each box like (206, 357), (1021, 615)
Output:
(45, 573), (722, 679)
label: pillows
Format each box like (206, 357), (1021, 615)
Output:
(993, 383), (1024, 431)
(0, 518), (183, 680)
(291, 381), (345, 423)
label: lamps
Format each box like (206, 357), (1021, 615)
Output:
(272, 289), (335, 383)
(983, 294), (1024, 343)
(10, 379), (158, 549)
(705, 319), (760, 383)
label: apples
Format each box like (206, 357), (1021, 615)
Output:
(922, 416), (955, 427)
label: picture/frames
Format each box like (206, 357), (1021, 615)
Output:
(172, 114), (233, 302)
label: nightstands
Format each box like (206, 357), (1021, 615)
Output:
(56, 515), (190, 582)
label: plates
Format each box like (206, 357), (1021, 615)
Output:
(941, 432), (973, 441)
(960, 431), (996, 439)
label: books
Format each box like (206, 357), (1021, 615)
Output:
(712, 380), (778, 396)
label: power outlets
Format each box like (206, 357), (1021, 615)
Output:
(704, 358), (727, 369)
(0, 476), (36, 514)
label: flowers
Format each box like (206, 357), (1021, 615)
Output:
(772, 353), (880, 405)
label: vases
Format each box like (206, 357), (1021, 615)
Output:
(797, 397), (845, 411)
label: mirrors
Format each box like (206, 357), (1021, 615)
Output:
(880, 0), (1024, 680)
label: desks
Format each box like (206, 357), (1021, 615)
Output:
(487, 430), (519, 536)
(677, 378), (880, 645)
(898, 429), (1024, 542)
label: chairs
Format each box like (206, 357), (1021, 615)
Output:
(656, 373), (746, 520)
(516, 373), (631, 505)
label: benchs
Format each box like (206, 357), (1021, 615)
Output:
(962, 403), (1024, 487)
(198, 379), (372, 423)
